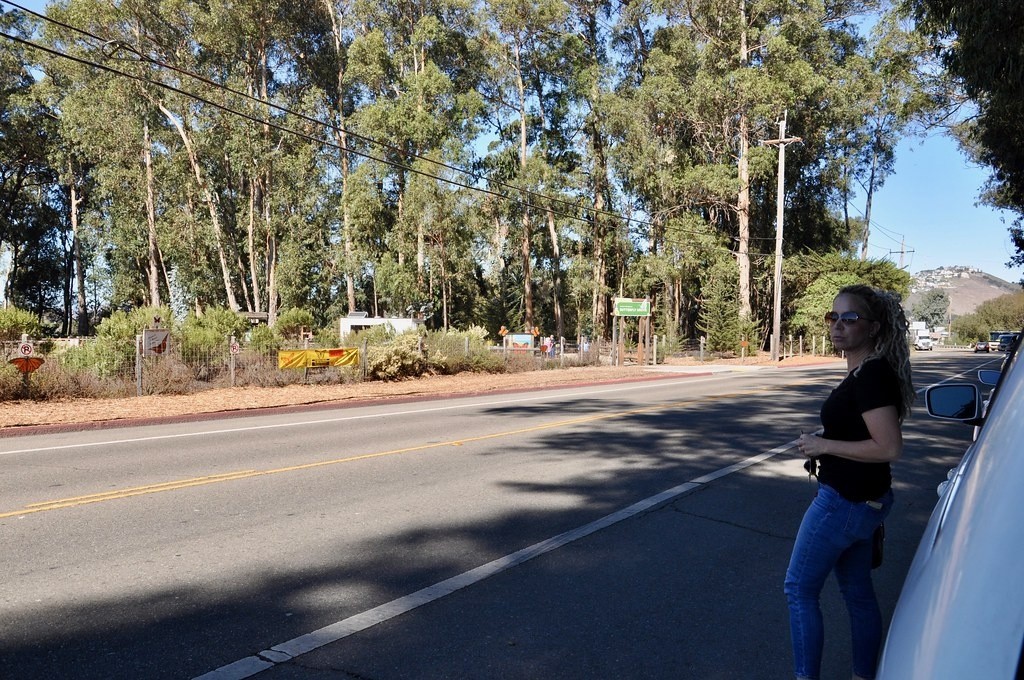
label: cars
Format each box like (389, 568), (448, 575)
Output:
(876, 327), (1022, 680)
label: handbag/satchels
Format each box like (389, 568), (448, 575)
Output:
(872, 521), (886, 570)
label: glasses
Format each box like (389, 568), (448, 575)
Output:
(824, 311), (873, 325)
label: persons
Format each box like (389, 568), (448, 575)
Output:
(784, 285), (918, 680)
